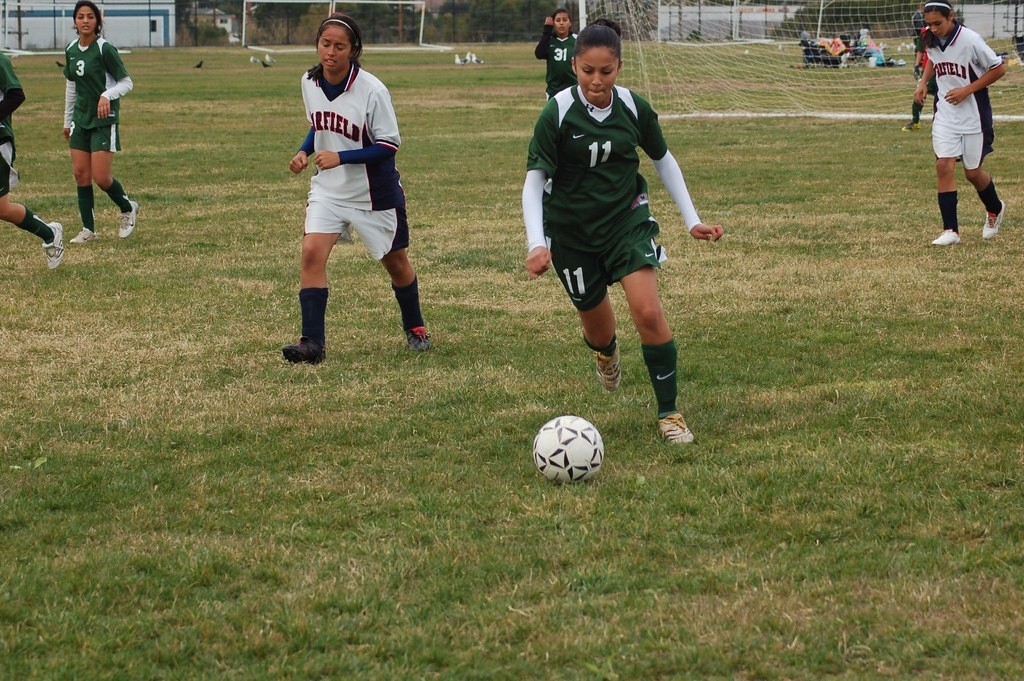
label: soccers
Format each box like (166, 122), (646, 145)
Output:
(530, 415), (605, 485)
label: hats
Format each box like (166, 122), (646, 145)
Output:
(801, 31), (810, 39)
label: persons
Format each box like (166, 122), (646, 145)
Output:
(902, 0), (1007, 247)
(0, 51), (63, 272)
(280, 13), (434, 364)
(799, 28), (885, 67)
(62, 0), (138, 245)
(519, 8), (727, 444)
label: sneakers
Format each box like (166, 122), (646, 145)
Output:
(593, 340), (622, 391)
(41, 222), (64, 270)
(658, 413), (693, 445)
(282, 336), (325, 365)
(931, 228), (960, 245)
(902, 121), (920, 133)
(405, 327), (430, 352)
(69, 228), (99, 244)
(119, 200), (138, 238)
(982, 199), (1005, 240)
